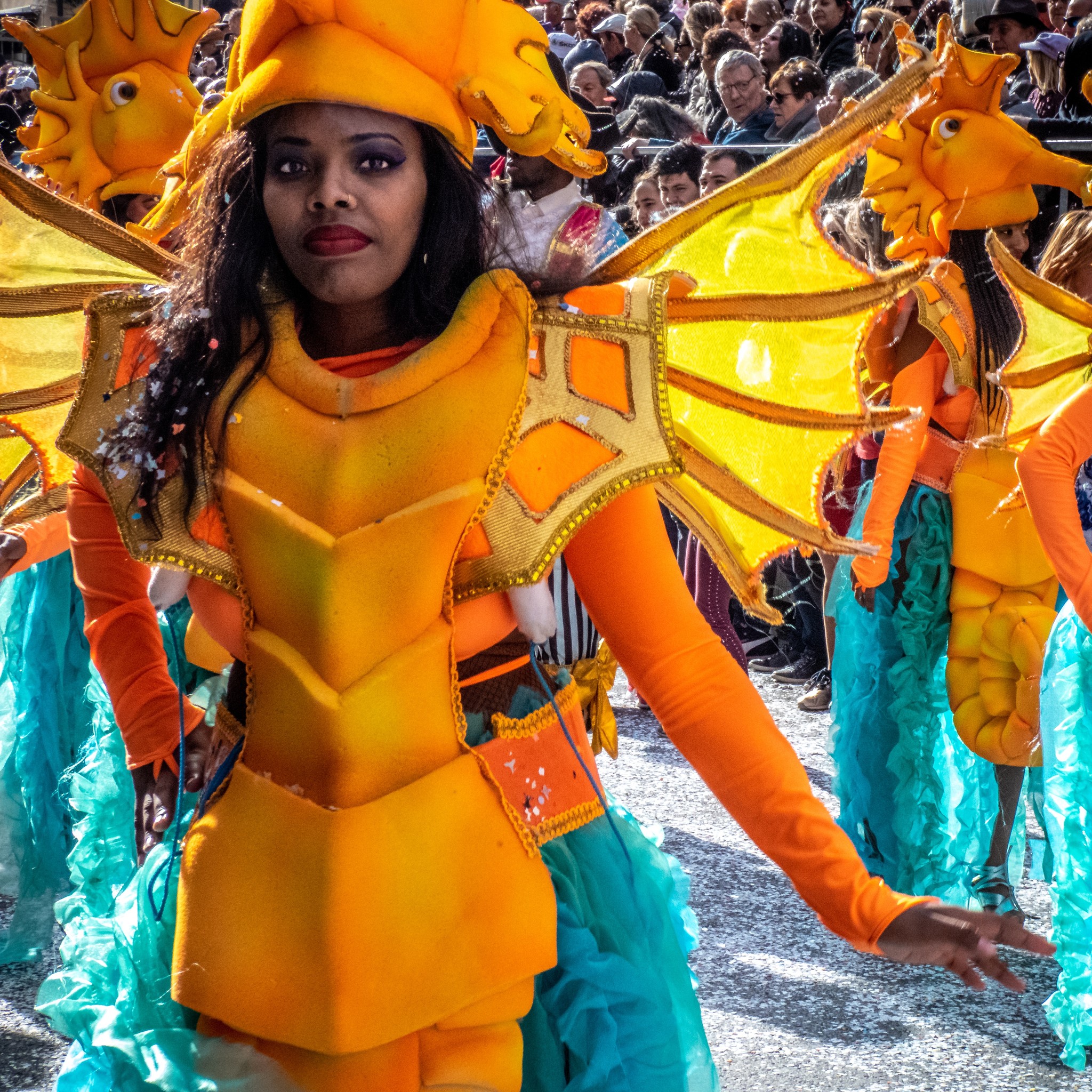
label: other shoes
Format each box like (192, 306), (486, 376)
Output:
(796, 668), (832, 711)
(803, 677), (817, 691)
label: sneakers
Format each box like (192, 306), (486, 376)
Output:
(748, 643), (805, 672)
(771, 654), (825, 684)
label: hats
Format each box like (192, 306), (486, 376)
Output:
(125, 0), (608, 241)
(2, 0), (221, 215)
(591, 14), (629, 33)
(861, 15), (1039, 262)
(1020, 32), (1072, 63)
(547, 33), (576, 58)
(534, 0), (567, 6)
(483, 44), (617, 158)
(975, 0), (1045, 36)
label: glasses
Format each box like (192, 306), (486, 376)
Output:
(854, 30), (882, 45)
(765, 91), (799, 105)
(1061, 15), (1083, 28)
(1056, 49), (1067, 66)
(717, 74), (757, 96)
(561, 16), (576, 21)
(740, 18), (769, 33)
(1048, 0), (1069, 6)
(884, 5), (914, 16)
(676, 42), (691, 49)
(621, 24), (630, 29)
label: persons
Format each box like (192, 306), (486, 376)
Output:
(68, 0), (1057, 1092)
(0, 1), (1092, 738)
(827, 14), (1092, 910)
(0, 1), (225, 970)
(1015, 362), (1092, 1071)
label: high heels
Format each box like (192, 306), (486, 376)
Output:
(966, 861), (1026, 922)
